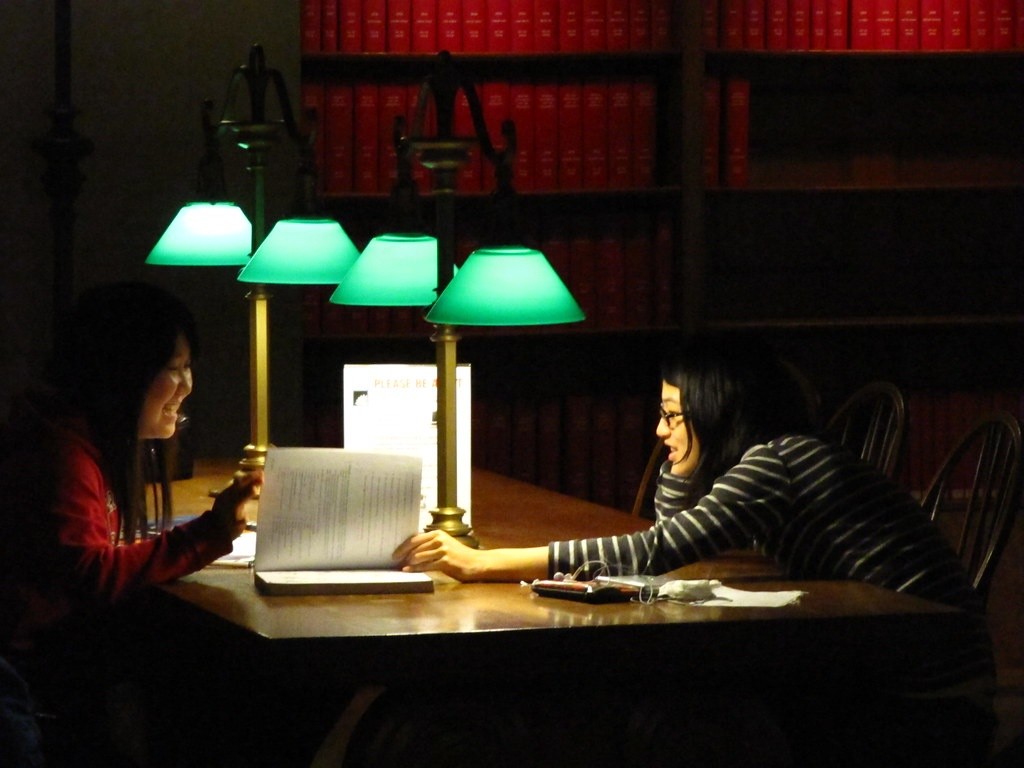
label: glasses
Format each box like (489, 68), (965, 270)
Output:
(658, 407), (683, 428)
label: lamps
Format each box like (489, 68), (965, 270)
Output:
(140, 44), (589, 571)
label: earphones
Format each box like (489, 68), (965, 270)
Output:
(553, 567), (583, 581)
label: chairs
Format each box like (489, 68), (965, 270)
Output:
(632, 360), (1024, 595)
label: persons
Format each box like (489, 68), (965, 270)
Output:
(389, 361), (999, 768)
(0, 282), (261, 768)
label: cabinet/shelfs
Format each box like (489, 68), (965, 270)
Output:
(299, 0), (1024, 342)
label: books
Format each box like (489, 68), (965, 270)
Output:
(471, 394), (646, 511)
(254, 447), (435, 596)
(120, 480), (257, 568)
(907, 391), (1024, 491)
(301, 0), (1024, 52)
(297, 78), (756, 336)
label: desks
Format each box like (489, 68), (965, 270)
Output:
(122, 466), (966, 768)
